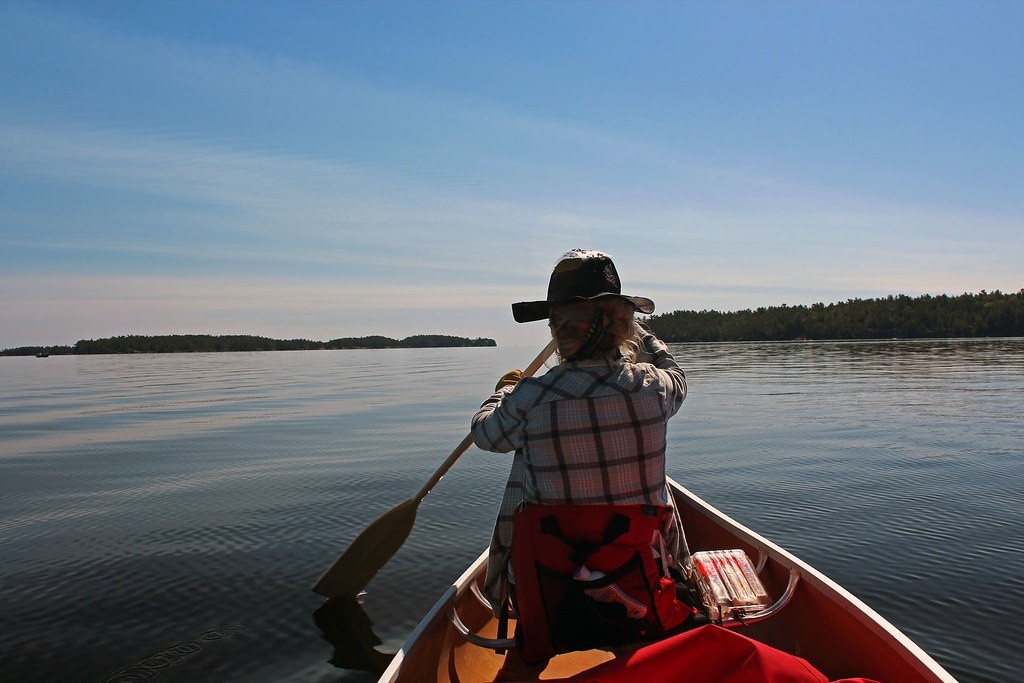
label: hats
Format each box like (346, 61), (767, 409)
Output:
(511, 249), (654, 324)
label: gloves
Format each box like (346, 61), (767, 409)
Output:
(495, 369), (523, 391)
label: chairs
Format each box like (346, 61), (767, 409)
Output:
(450, 497), (799, 654)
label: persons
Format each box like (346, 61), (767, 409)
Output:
(471, 268), (687, 618)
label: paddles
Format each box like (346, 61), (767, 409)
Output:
(308, 333), (562, 603)
